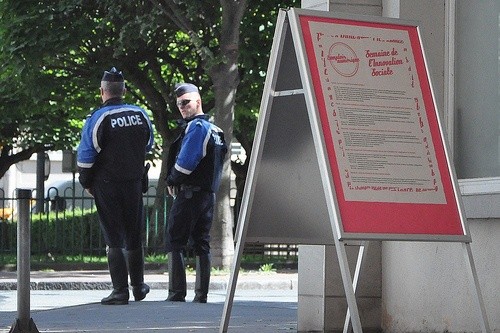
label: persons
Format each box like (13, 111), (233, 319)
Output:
(164, 83), (227, 303)
(77, 67), (154, 305)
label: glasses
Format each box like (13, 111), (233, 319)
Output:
(177, 100), (191, 105)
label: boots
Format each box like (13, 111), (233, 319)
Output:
(101, 247), (129, 305)
(128, 250), (150, 301)
(192, 256), (210, 303)
(165, 252), (187, 302)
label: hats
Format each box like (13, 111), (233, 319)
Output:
(174, 83), (198, 96)
(102, 67), (124, 81)
